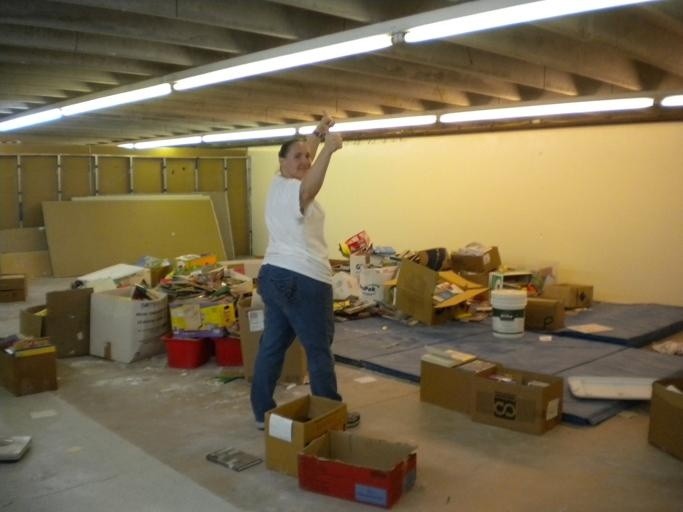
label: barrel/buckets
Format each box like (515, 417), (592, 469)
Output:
(489, 288), (528, 339)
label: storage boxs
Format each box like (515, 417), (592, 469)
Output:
(0, 332), (55, 397)
(0, 273), (27, 304)
(297, 431), (417, 508)
(419, 352), (501, 415)
(265, 392), (348, 478)
(648, 369), (683, 459)
(470, 365), (565, 436)
(18, 252), (306, 384)
(348, 240), (594, 334)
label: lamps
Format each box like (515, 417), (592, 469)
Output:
(205, 122), (297, 144)
(173, 32), (397, 91)
(397, 0), (651, 47)
(0, 108), (62, 133)
(299, 110), (439, 137)
(438, 94), (652, 125)
(62, 82), (172, 117)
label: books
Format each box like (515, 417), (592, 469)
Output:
(0, 334), (56, 357)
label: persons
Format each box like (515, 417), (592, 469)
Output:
(251, 113), (361, 431)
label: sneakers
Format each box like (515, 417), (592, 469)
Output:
(345, 412), (359, 428)
(256, 421), (264, 430)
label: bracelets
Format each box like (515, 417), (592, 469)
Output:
(313, 131), (323, 138)
